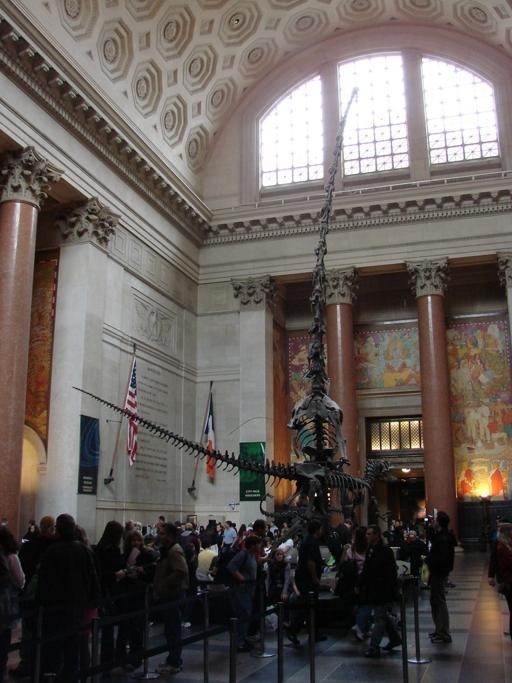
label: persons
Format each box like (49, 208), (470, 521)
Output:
(174, 511), (457, 656)
(464, 397), (508, 446)
(1, 511), (187, 682)
(487, 515), (511, 638)
(489, 513), (503, 552)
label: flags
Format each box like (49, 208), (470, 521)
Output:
(203, 390), (214, 483)
(126, 356), (137, 467)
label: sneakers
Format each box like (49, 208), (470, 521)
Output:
(351, 624), (365, 641)
(155, 662), (184, 674)
(382, 638), (402, 650)
(428, 632), (453, 644)
(360, 647), (381, 655)
